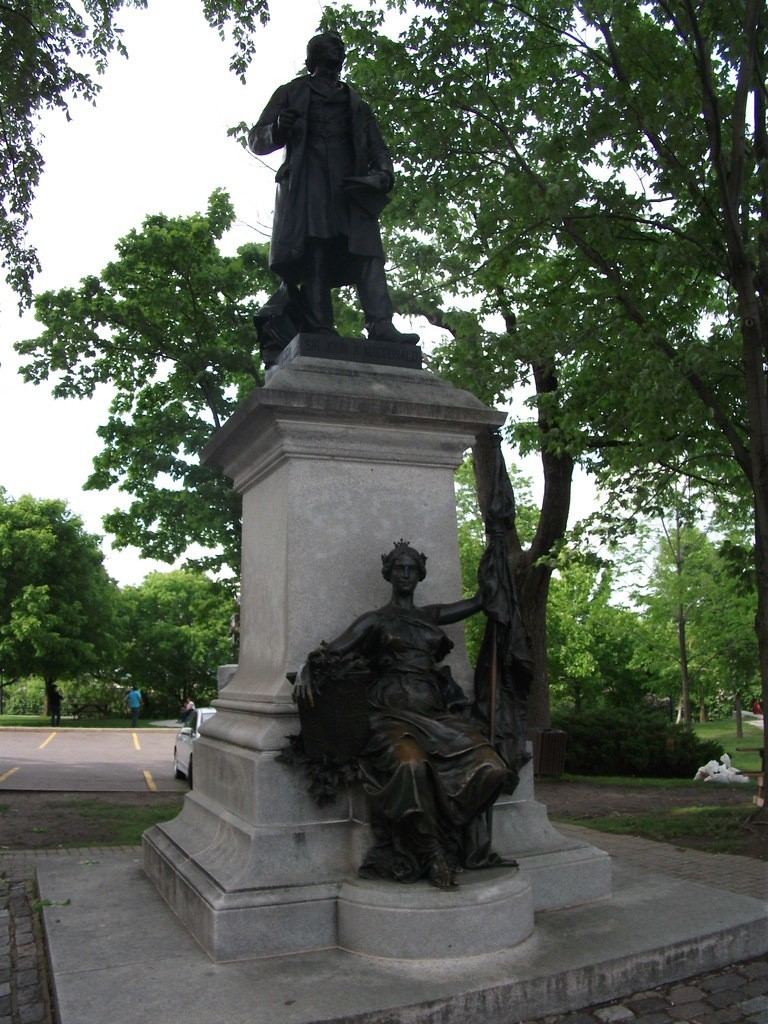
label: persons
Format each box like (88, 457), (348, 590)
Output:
(275, 539), (530, 894)
(48, 684), (63, 726)
(248, 29), (421, 346)
(125, 686), (144, 729)
(178, 697), (195, 722)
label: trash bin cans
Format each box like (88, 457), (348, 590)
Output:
(538, 726), (569, 777)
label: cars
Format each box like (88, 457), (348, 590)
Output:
(173, 707), (217, 790)
